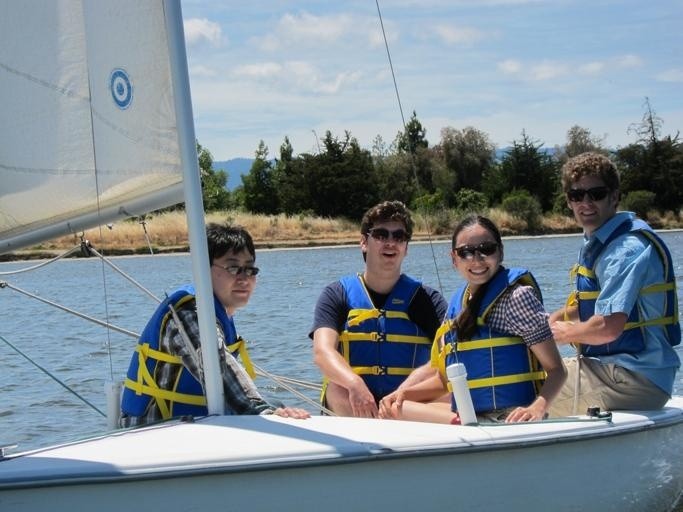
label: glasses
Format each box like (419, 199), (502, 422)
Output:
(367, 228), (411, 242)
(212, 265), (259, 276)
(567, 187), (607, 201)
(454, 241), (499, 259)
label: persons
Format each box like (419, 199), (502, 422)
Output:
(120, 222), (312, 429)
(546, 151), (682, 418)
(307, 200), (449, 418)
(378, 215), (570, 425)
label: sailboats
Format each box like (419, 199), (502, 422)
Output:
(1, 2), (683, 510)
